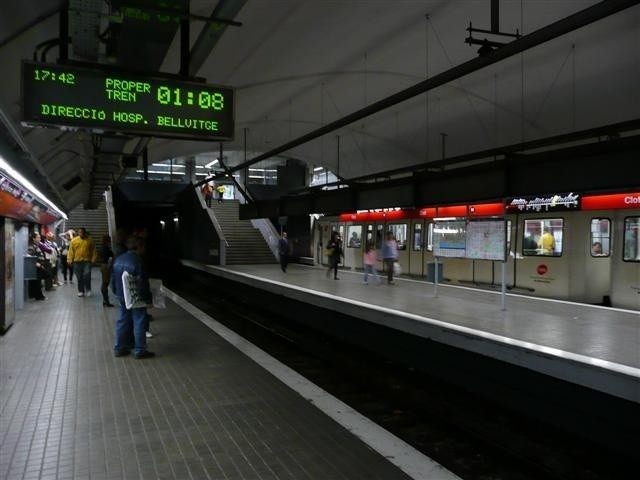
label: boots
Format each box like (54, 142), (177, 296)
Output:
(101, 287), (115, 308)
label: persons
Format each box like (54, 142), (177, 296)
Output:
(278, 232), (291, 272)
(538, 226), (555, 257)
(217, 184), (226, 205)
(363, 239), (382, 286)
(202, 181), (215, 208)
(26, 223), (157, 360)
(380, 231), (399, 284)
(349, 231), (361, 247)
(326, 232), (344, 281)
(592, 242), (608, 256)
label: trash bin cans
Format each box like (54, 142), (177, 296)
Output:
(24, 256), (38, 281)
(426, 261), (443, 283)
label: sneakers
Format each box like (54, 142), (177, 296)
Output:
(134, 350), (156, 359)
(145, 332), (153, 338)
(77, 292), (92, 297)
(113, 350), (132, 358)
(325, 273), (340, 281)
(36, 281), (74, 301)
(361, 278), (397, 288)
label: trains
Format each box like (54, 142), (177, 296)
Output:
(274, 190), (640, 314)
